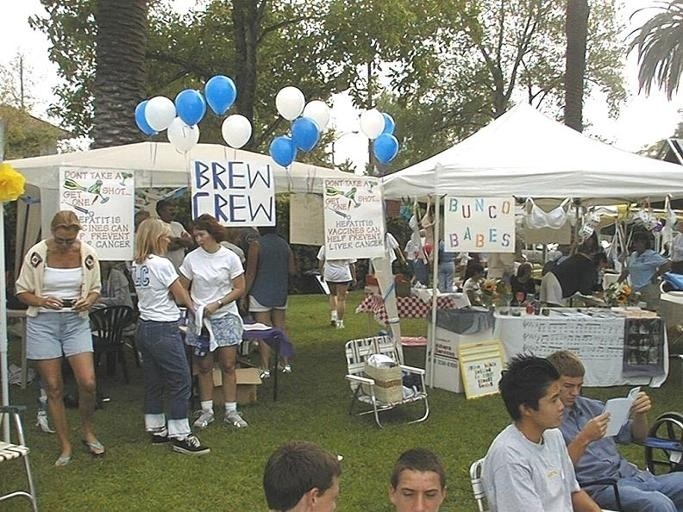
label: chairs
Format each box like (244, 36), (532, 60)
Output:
(79, 301), (136, 411)
(341, 332), (431, 431)
(468, 451), (619, 511)
(0, 402), (38, 512)
(565, 426), (683, 511)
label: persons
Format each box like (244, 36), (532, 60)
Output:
(387, 447), (447, 512)
(481, 350), (601, 511)
(130, 218), (210, 456)
(261, 441), (341, 512)
(386, 220), (682, 310)
(316, 243), (357, 330)
(88, 199), (258, 372)
(239, 226), (294, 377)
(14, 211), (106, 467)
(545, 352), (681, 511)
(177, 214), (249, 429)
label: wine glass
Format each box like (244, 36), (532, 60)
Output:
(515, 292), (524, 312)
(505, 293), (513, 312)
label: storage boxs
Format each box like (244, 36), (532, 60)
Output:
(191, 359), (263, 409)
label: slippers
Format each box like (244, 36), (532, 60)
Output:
(54, 456), (72, 468)
(82, 435), (106, 455)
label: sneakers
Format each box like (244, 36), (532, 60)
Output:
(192, 407), (216, 429)
(330, 315), (337, 327)
(224, 410), (249, 429)
(336, 320), (345, 330)
(170, 433), (211, 457)
(151, 429), (177, 446)
(258, 369), (272, 380)
(272, 361), (293, 374)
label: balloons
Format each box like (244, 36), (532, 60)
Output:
(134, 74), (397, 168)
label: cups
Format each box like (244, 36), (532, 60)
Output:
(526, 294), (535, 305)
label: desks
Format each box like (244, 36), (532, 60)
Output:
(178, 315), (286, 414)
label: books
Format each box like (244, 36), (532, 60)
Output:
(600, 385), (641, 438)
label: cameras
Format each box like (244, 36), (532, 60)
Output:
(60, 299), (77, 307)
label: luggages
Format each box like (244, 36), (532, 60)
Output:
(395, 275), (411, 297)
